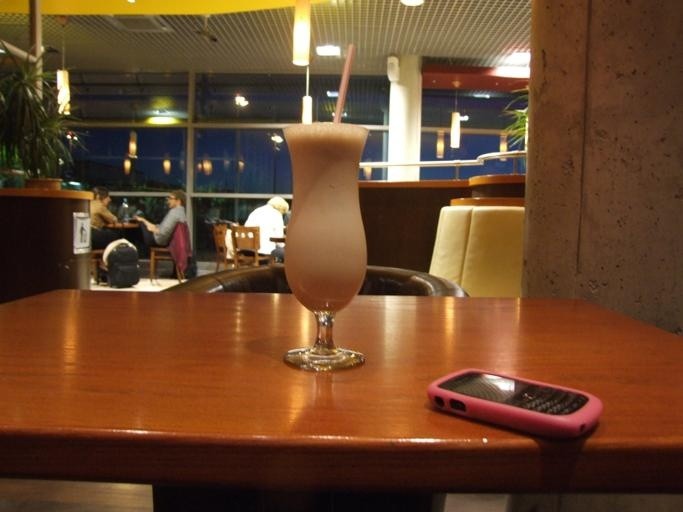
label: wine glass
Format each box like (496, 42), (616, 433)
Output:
(281, 121), (370, 373)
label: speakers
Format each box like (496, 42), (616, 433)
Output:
(387, 56), (400, 82)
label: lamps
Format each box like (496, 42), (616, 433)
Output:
(146, 111), (179, 125)
(56, 40), (72, 116)
(449, 91), (460, 147)
(233, 96), (250, 107)
(316, 45), (341, 57)
(122, 127), (508, 181)
(401, 1), (425, 6)
(327, 91), (338, 97)
(291, 1), (313, 124)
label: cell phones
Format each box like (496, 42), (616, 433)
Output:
(424, 366), (605, 440)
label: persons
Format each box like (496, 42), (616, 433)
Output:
(239, 196), (290, 265)
(89, 186), (119, 287)
(132, 188), (186, 259)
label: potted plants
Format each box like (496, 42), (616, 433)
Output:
(0, 39), (89, 190)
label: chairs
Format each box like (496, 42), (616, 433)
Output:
(153, 266), (471, 512)
(90, 218), (286, 289)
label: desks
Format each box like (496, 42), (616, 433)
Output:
(0, 288), (683, 512)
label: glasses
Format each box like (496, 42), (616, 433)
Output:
(166, 197), (176, 201)
